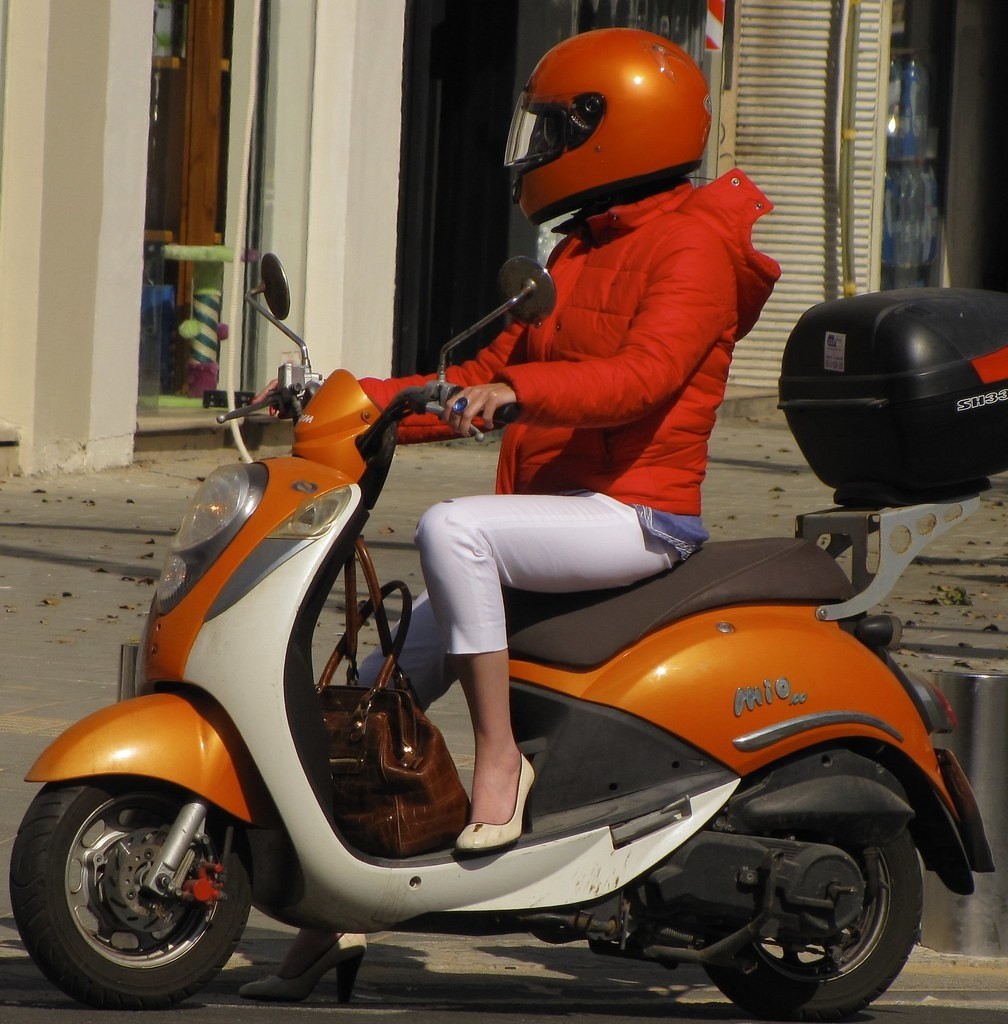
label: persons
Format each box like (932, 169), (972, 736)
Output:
(241, 30), (780, 1001)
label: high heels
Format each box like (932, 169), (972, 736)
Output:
(455, 746), (540, 860)
(236, 932), (371, 1001)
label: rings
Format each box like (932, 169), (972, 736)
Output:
(451, 396), (469, 414)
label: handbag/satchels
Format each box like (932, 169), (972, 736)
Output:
(314, 533), (471, 857)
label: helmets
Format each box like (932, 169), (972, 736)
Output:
(502, 24), (713, 227)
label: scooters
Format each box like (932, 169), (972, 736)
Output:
(6, 242), (1008, 1023)
